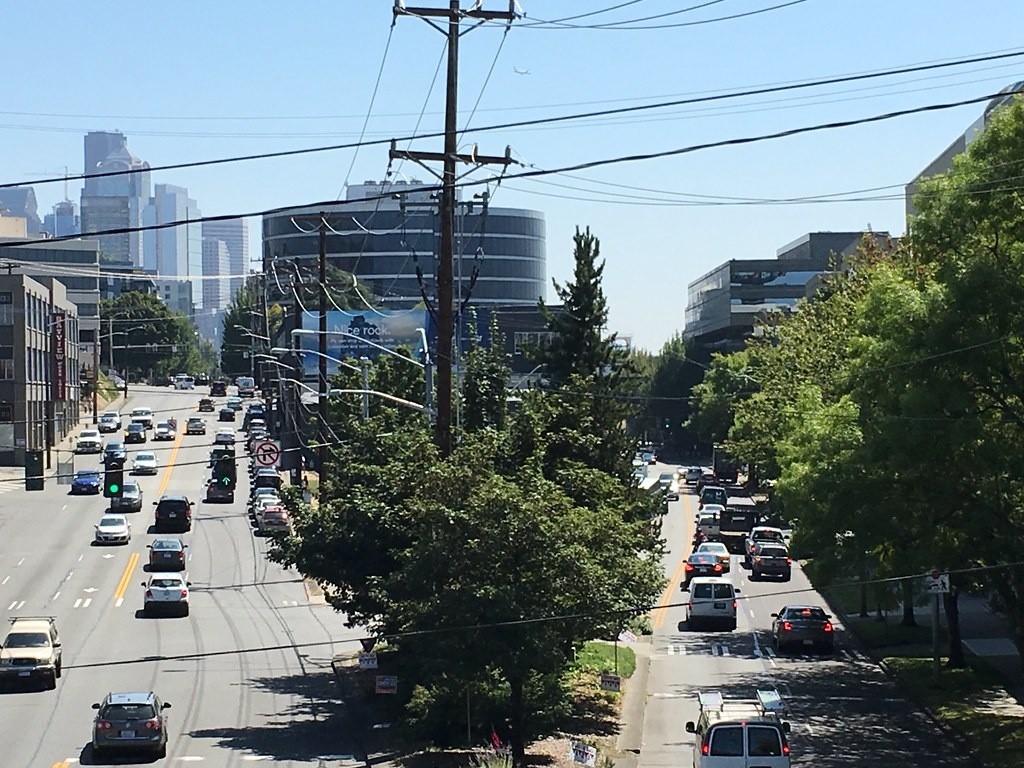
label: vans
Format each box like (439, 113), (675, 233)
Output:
(686, 710), (791, 768)
(681, 576), (742, 630)
(129, 408), (154, 428)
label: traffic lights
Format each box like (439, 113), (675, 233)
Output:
(104, 456), (124, 498)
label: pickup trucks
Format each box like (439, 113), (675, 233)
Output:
(742, 527), (789, 560)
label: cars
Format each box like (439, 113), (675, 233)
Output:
(111, 480), (143, 512)
(695, 503), (725, 537)
(153, 423), (174, 440)
(659, 471), (680, 501)
(683, 542), (730, 577)
(72, 471), (102, 494)
(215, 427), (235, 444)
(94, 514), (131, 545)
(205, 445), (238, 502)
(97, 412), (123, 433)
(124, 423), (147, 444)
(200, 399), (215, 411)
(771, 605), (834, 651)
(141, 573), (192, 617)
(186, 418), (208, 435)
(146, 538), (188, 571)
(131, 451), (159, 474)
(247, 402), (290, 536)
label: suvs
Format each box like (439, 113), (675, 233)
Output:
(91, 691), (171, 759)
(678, 466), (729, 510)
(152, 496), (194, 532)
(750, 542), (792, 580)
(0, 616), (62, 692)
(76, 430), (105, 453)
(210, 381), (227, 397)
